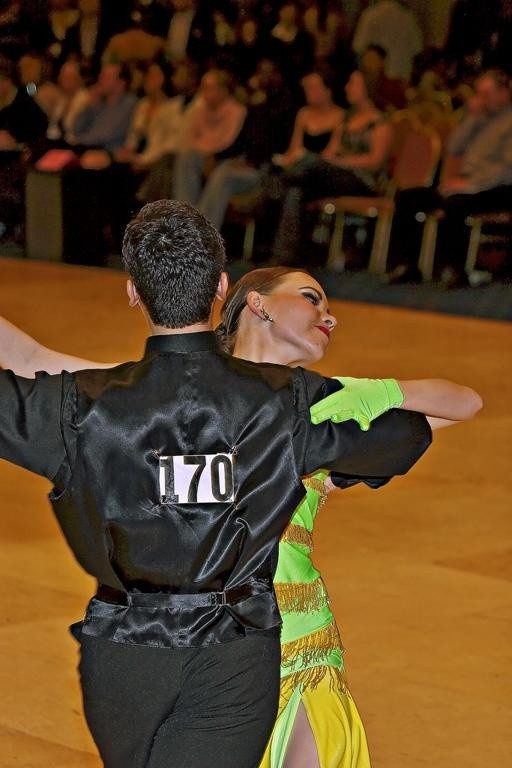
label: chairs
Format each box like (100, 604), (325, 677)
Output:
(319, 118), (512, 289)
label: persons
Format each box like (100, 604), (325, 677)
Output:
(2, 200), (434, 767)
(1, 1), (511, 287)
(2, 267), (484, 766)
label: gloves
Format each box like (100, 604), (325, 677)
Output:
(310, 376), (404, 431)
